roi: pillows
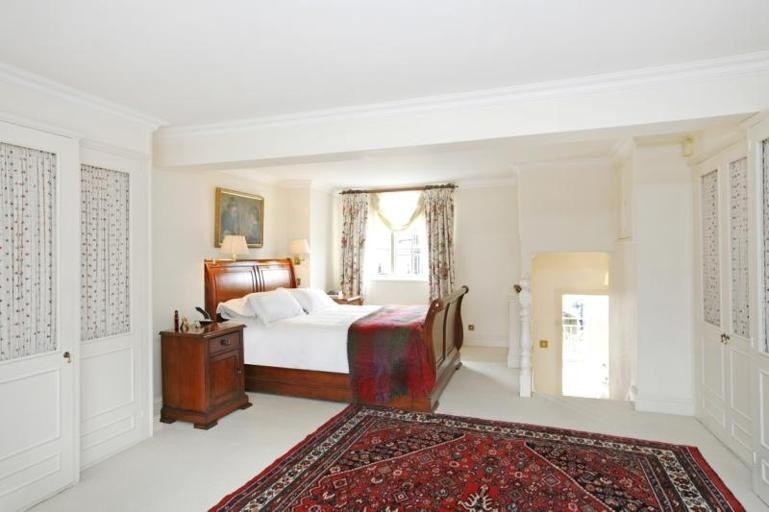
[216,286,338,325]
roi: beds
[204,258,468,412]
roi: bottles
[174,310,189,332]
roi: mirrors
[562,294,610,402]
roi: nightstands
[161,324,249,427]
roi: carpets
[207,401,747,512]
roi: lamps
[288,237,312,265]
[219,234,250,262]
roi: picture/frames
[215,188,264,251]
